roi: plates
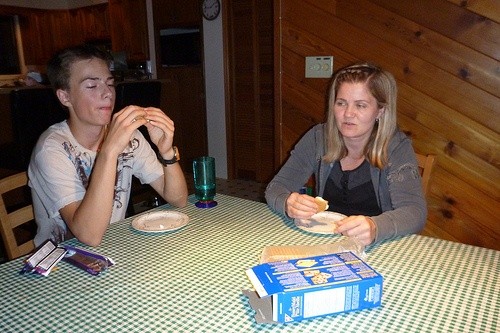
[294,210,349,234]
[131,210,190,233]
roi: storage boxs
[241,250,383,326]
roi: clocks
[200,0,221,21]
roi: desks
[0,79,162,231]
[0,191,500,333]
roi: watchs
[157,146,180,167]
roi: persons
[27,46,188,249]
[265,63,428,247]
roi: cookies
[260,242,359,265]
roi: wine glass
[193,156,218,209]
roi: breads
[313,196,329,213]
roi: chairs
[0,171,39,261]
[412,152,434,197]
